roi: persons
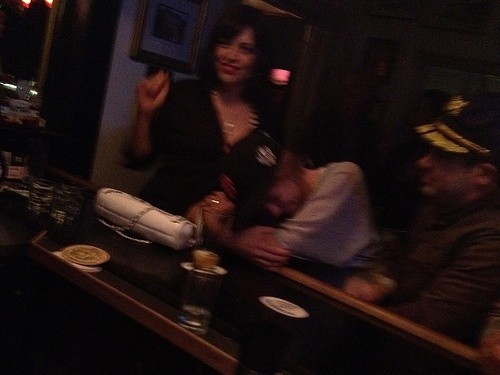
[188,150,396,282]
[120,0,311,245]
[344,96,500,350]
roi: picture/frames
[127,0,210,73]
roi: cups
[194,250,219,270]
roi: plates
[63,243,110,265]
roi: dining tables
[0,171,488,375]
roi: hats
[220,129,281,233]
[410,90,500,156]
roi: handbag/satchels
[92,187,204,250]
